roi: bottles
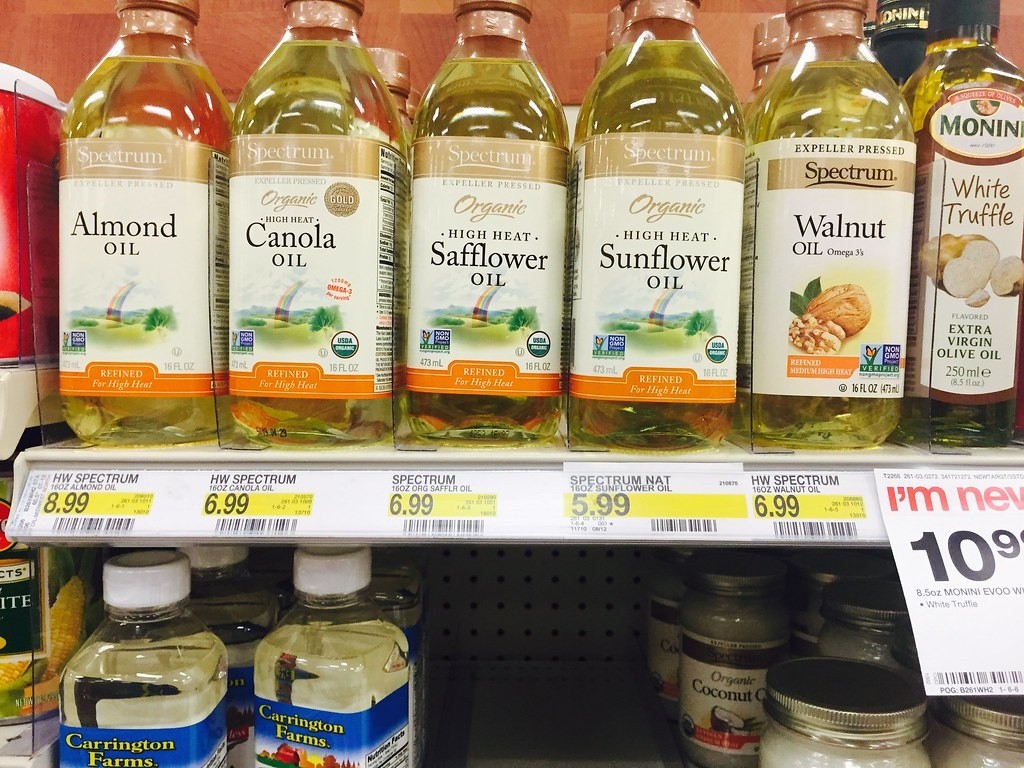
[61,0,1024,768]
